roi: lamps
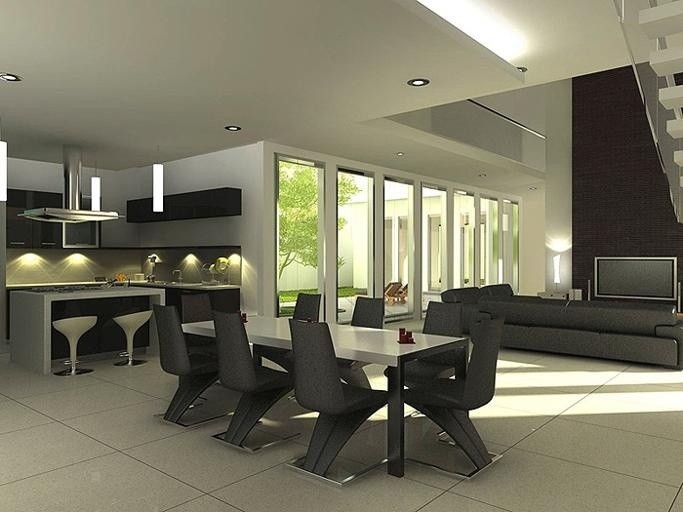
[551,254,562,285]
[152,144,164,212]
[90,159,101,212]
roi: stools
[50,314,99,379]
[110,308,155,368]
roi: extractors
[17,141,125,223]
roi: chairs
[267,293,323,361]
[285,316,388,486]
[383,281,409,305]
[150,304,235,433]
[400,313,506,481]
[338,297,385,389]
[211,311,303,459]
[384,302,464,435]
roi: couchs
[420,284,683,370]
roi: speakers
[574,290,582,300]
[569,289,575,300]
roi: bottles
[237,310,248,324]
[399,327,413,342]
[115,273,123,281]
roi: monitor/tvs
[594,256,678,301]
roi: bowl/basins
[133,274,145,281]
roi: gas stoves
[31,283,105,291]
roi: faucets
[171,269,183,283]
[107,278,118,289]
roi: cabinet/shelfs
[5,188,63,248]
[172,187,241,220]
[126,194,171,223]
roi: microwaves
[61,218,99,249]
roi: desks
[8,288,166,376]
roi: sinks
[169,280,203,287]
